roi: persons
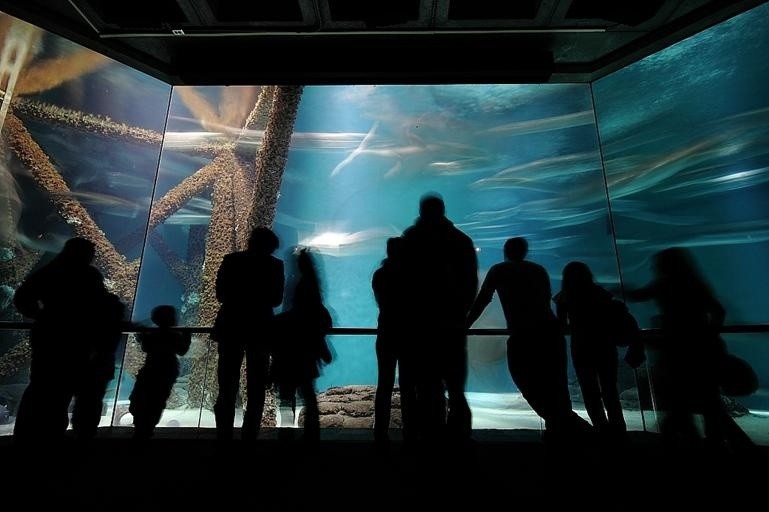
[369,235,411,444]
[70,265,126,435]
[551,262,629,441]
[464,237,597,445]
[398,191,480,454]
[279,248,323,444]
[11,237,99,438]
[609,241,755,446]
[126,304,194,436]
[209,226,288,445]
